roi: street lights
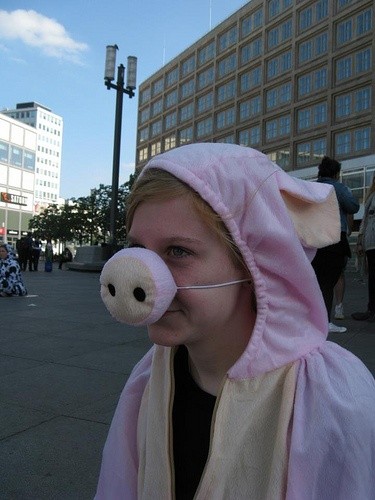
[104,44,138,258]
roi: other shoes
[352,312,373,321]
[335,303,344,319]
[328,322,347,333]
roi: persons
[44,239,55,272]
[353,174,375,321]
[59,247,73,269]
[333,274,345,319]
[0,243,28,296]
[95,140,374,500]
[16,232,42,271]
[311,159,359,333]
